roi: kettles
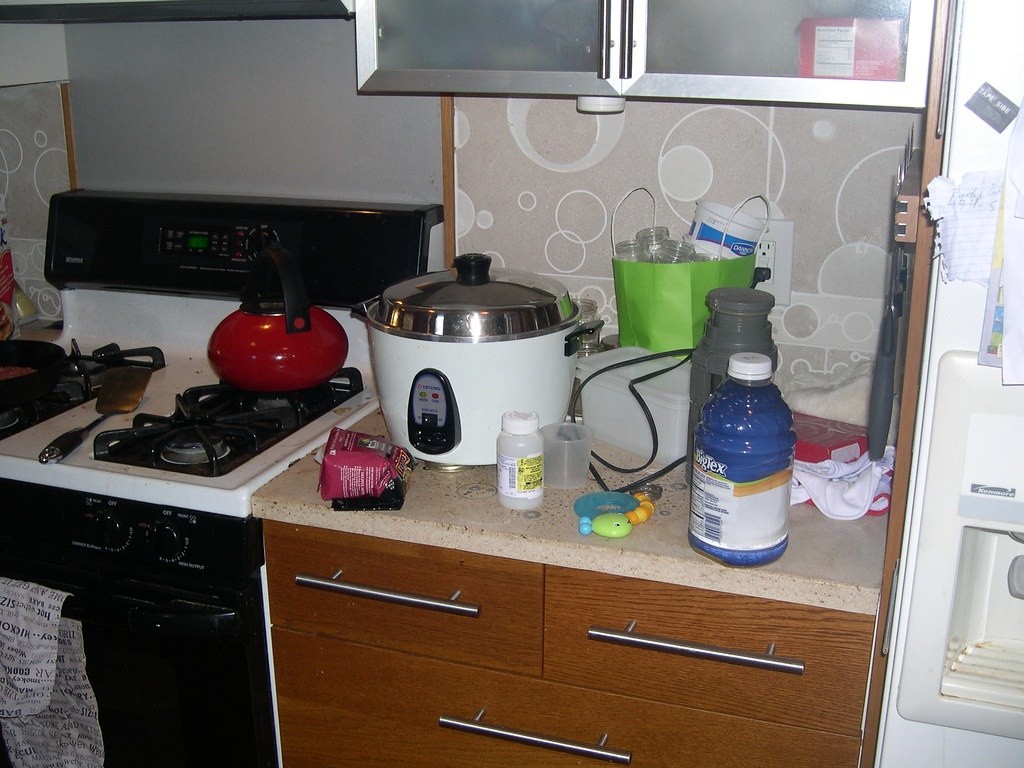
[207,248,349,392]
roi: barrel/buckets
[684,201,768,261]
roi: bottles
[688,352,797,567]
[496,410,544,509]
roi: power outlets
[754,219,796,306]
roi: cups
[615,227,720,265]
[570,298,600,344]
[567,343,604,416]
[540,422,592,489]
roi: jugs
[685,288,777,484]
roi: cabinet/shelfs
[353,0,937,109]
[264,519,876,768]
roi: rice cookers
[350,254,604,473]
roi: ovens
[0,478,278,768]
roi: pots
[0,340,65,403]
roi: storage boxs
[798,18,902,81]
[792,412,869,463]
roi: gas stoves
[0,188,441,518]
